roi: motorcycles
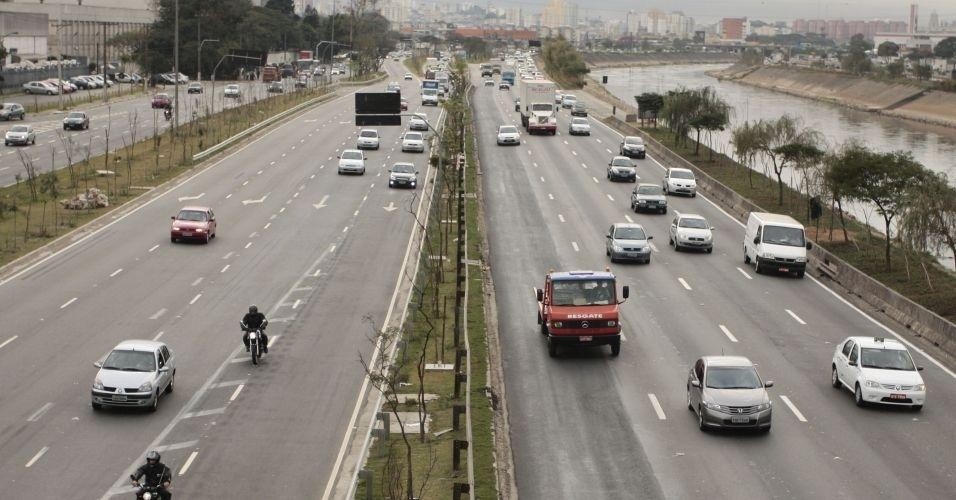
[164,105,171,120]
[238,315,269,365]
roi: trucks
[533,265,632,362]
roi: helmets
[146,449,160,469]
[248,305,257,314]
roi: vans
[743,210,812,278]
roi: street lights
[314,41,339,62]
[95,30,101,73]
[0,31,18,39]
[207,51,263,123]
[55,20,78,112]
[70,31,80,61]
[194,38,222,81]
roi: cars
[5,58,81,73]
[1,101,25,123]
[831,333,929,412]
[150,93,173,109]
[426,52,450,72]
[662,167,699,198]
[670,214,717,254]
[336,71,451,189]
[607,223,654,265]
[629,182,667,217]
[619,133,646,158]
[187,80,203,94]
[90,339,176,410]
[686,354,774,436]
[384,48,411,63]
[63,111,89,132]
[223,83,241,97]
[607,155,636,186]
[170,204,217,246]
[260,54,346,93]
[4,124,37,148]
[477,47,591,144]
[21,70,190,94]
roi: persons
[164,102,172,118]
[256,69,260,79]
[239,304,268,354]
[590,280,610,302]
